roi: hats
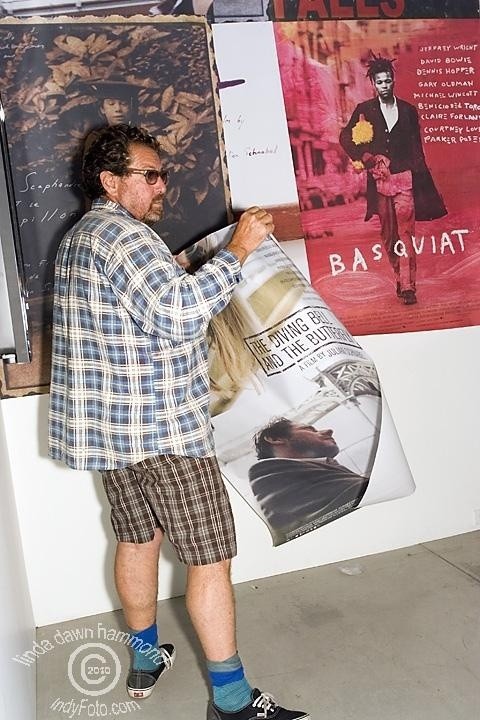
[75,68,148,96]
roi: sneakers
[404,292,417,305]
[208,688,311,719]
[123,642,177,701]
[395,282,402,296]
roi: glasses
[123,164,170,185]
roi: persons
[248,416,369,547]
[338,49,449,304]
[45,122,312,720]
[175,234,257,418]
[76,73,147,125]
